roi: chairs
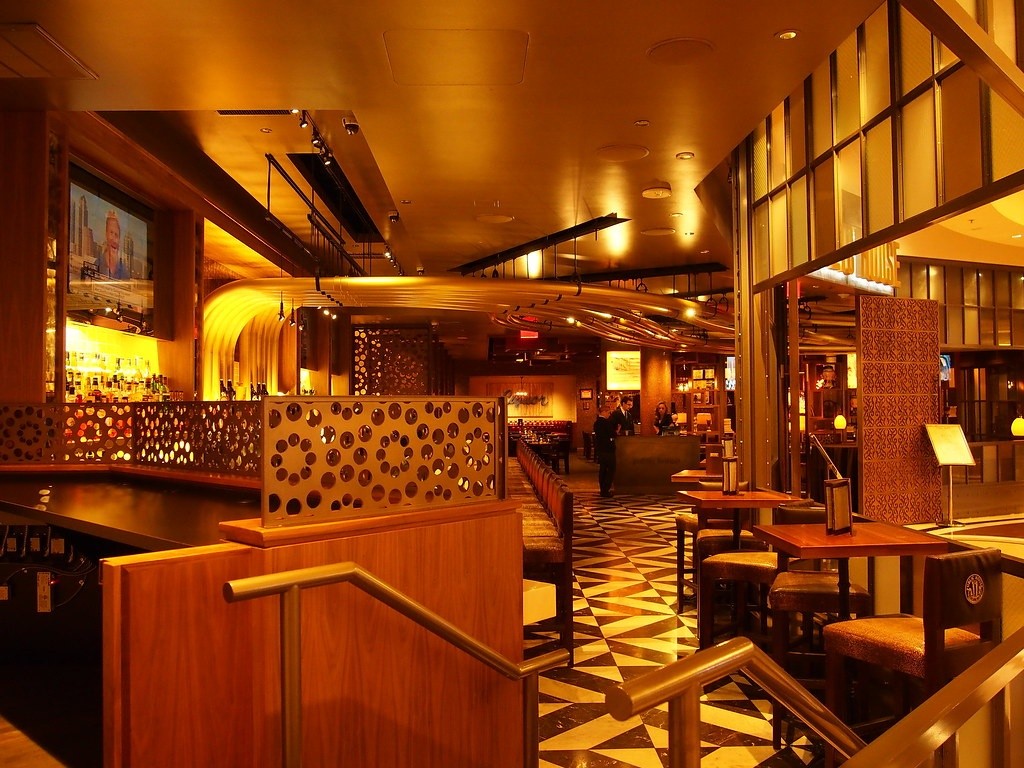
[551,440,570,475]
[582,432,599,463]
[675,481,1004,768]
[507,438,575,667]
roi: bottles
[64,351,184,404]
[233,382,246,401]
[251,382,257,401]
[261,383,268,396]
[220,379,226,400]
[225,380,236,401]
[303,386,316,396]
[256,382,262,401]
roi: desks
[528,441,554,465]
[750,523,949,726]
[677,488,812,643]
[546,434,569,442]
[670,470,723,483]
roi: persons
[517,420,523,430]
[93,217,130,280]
[653,401,674,436]
[610,397,634,430]
[593,404,621,498]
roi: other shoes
[600,490,614,497]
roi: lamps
[676,413,687,426]
[833,415,847,430]
[291,108,332,166]
[278,257,305,331]
[1011,418,1024,436]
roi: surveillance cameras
[341,117,360,134]
[417,266,425,275]
[387,210,398,223]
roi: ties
[625,411,626,421]
